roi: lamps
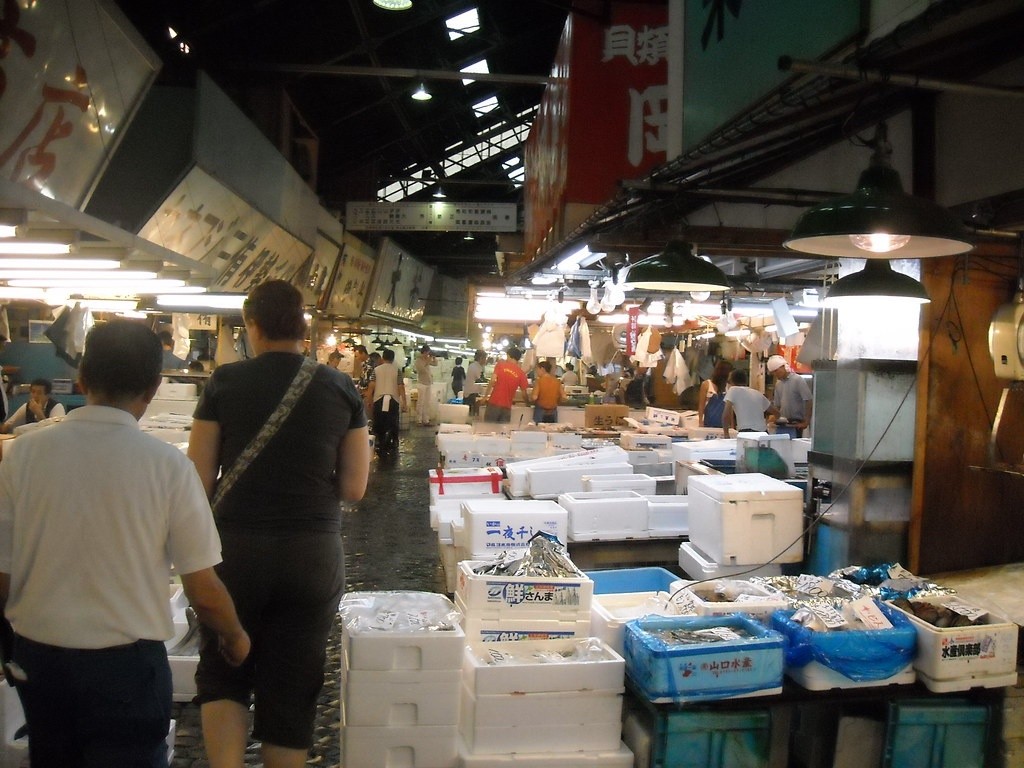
[0,279,212,301]
[369,0,413,13]
[617,210,740,298]
[410,80,433,102]
[0,260,168,280]
[782,130,980,265]
[432,183,447,197]
[826,258,932,304]
[0,227,85,253]
[553,234,608,274]
[0,269,193,289]
[0,246,135,272]
[0,207,33,239]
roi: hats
[767,355,791,372]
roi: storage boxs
[0,382,1024,768]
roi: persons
[451,357,466,398]
[587,366,606,393]
[186,281,370,768]
[529,361,566,425]
[464,350,488,416]
[326,344,383,446]
[0,318,250,768]
[479,348,530,421]
[561,363,579,386]
[366,350,407,448]
[415,345,438,427]
[157,330,188,368]
[171,360,210,396]
[699,355,813,439]
[0,378,65,433]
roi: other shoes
[417,424,422,426]
[424,423,437,426]
